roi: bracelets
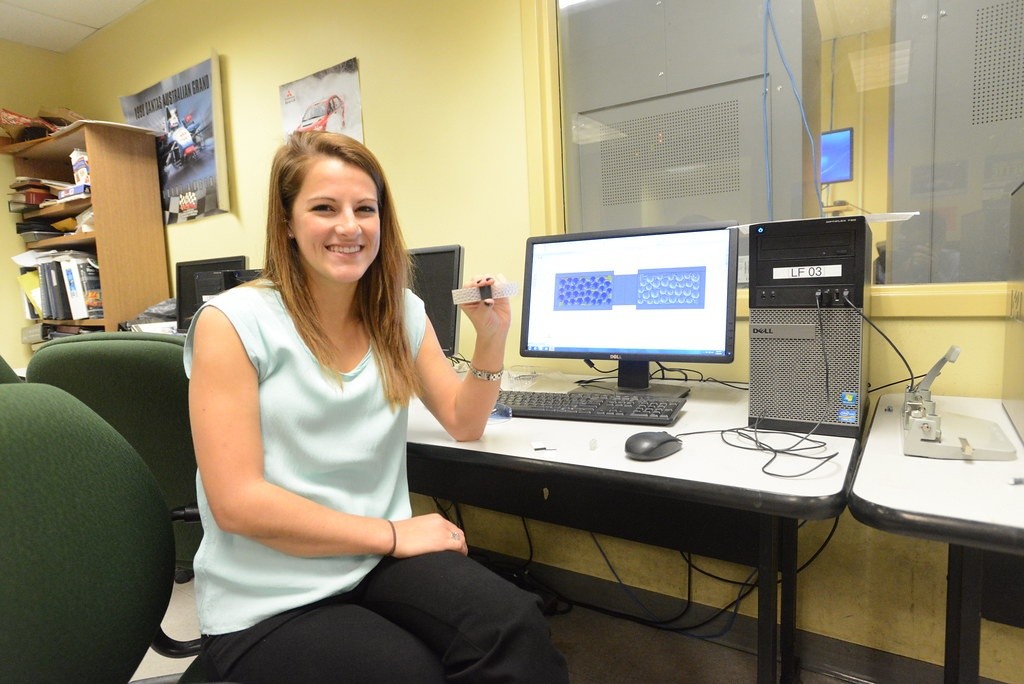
[468,362,508,382]
[383,519,398,556]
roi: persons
[178,126,572,683]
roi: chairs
[0,382,208,684]
[0,331,205,583]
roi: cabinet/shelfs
[11,122,171,352]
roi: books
[77,262,105,320]
[8,178,64,215]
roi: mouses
[625,430,683,461]
[834,201,848,206]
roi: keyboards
[493,390,688,426]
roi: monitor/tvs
[407,244,465,359]
[176,255,246,333]
[820,127,854,184]
[519,220,740,398]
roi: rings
[450,531,459,541]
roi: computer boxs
[196,269,265,309]
[749,216,873,439]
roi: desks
[848,393,1024,684]
[407,368,871,684]
